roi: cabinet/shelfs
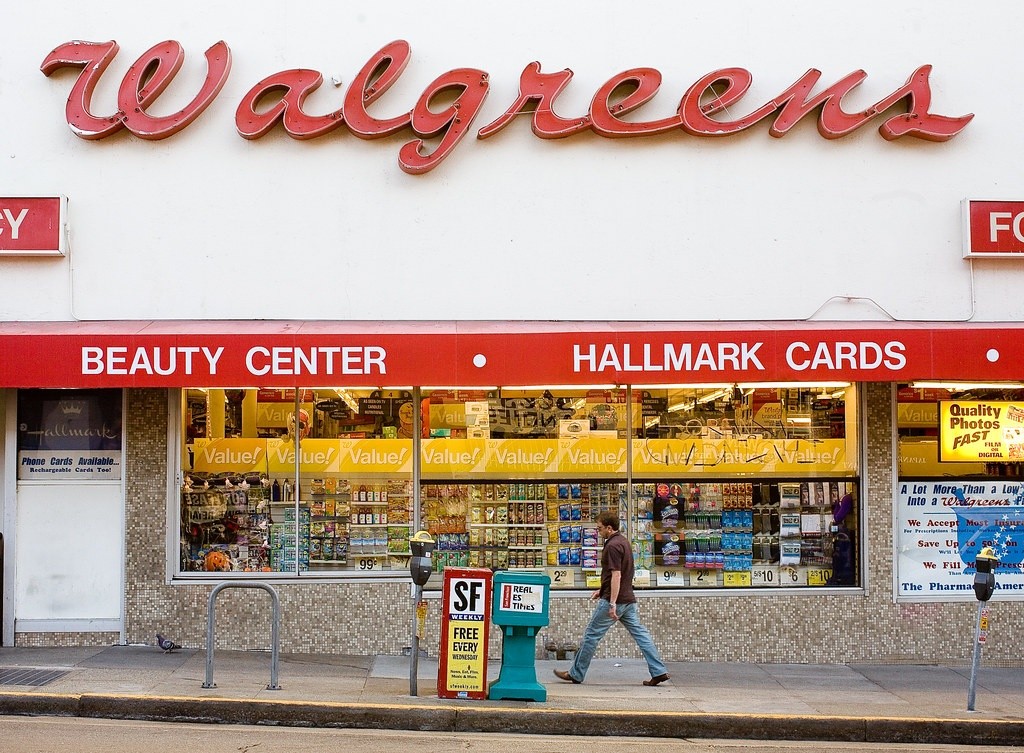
[348,478,654,572]
[309,493,351,563]
[683,482,854,572]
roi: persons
[553,512,671,685]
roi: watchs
[608,603,616,608]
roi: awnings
[0,319,1024,389]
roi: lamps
[668,389,733,413]
[817,387,832,399]
[381,381,852,390]
[910,380,1024,389]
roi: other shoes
[553,669,581,684]
[643,673,670,686]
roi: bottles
[373,482,381,502]
[359,483,367,502]
[352,483,359,502]
[380,483,388,501]
[283,478,290,502]
[273,478,280,501]
[367,483,373,502]
[293,480,300,501]
[351,506,388,525]
[350,528,387,554]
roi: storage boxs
[352,514,387,524]
[326,498,336,516]
[696,553,706,568]
[557,419,617,440]
[465,402,490,439]
[706,553,715,568]
[900,435,985,476]
[430,429,466,439]
[382,426,397,439]
[898,403,939,428]
[429,404,466,428]
[353,491,387,501]
[256,390,316,427]
[337,432,369,438]
[325,477,336,494]
[701,426,763,441]
[270,508,310,572]
[686,552,696,568]
[715,553,724,568]
